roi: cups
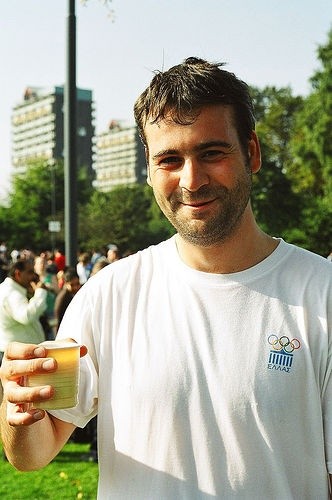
[22,340,80,410]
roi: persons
[0,245,123,462]
[0,57,332,500]
